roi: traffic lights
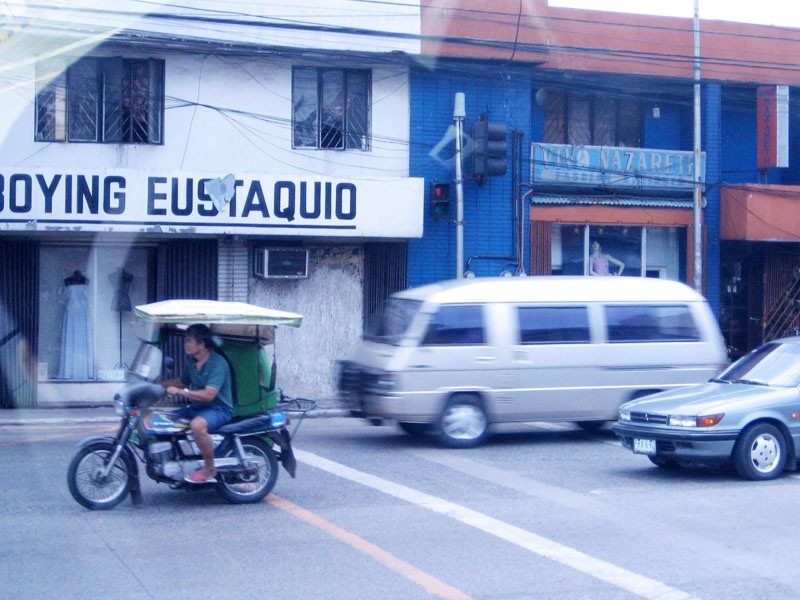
[431,181,451,219]
[473,113,509,177]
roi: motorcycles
[66,298,316,511]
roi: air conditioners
[254,245,309,279]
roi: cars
[612,336,800,480]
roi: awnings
[720,184,800,242]
[529,194,706,226]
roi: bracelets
[184,387,189,396]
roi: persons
[217,338,275,447]
[590,239,625,276]
[301,104,366,148]
[157,325,233,484]
[603,130,626,147]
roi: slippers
[184,471,218,483]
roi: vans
[335,275,727,449]
